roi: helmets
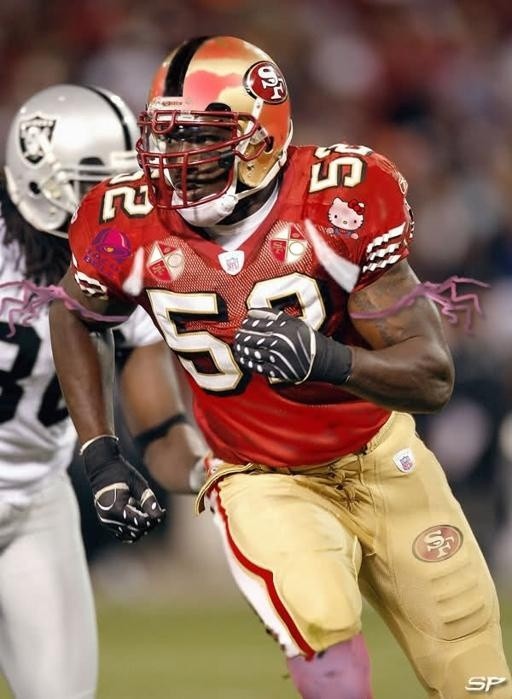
[3,82,140,240]
[134,34,294,228]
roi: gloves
[233,308,350,387]
[80,434,168,545]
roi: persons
[0,80,222,699]
[50,34,512,699]
